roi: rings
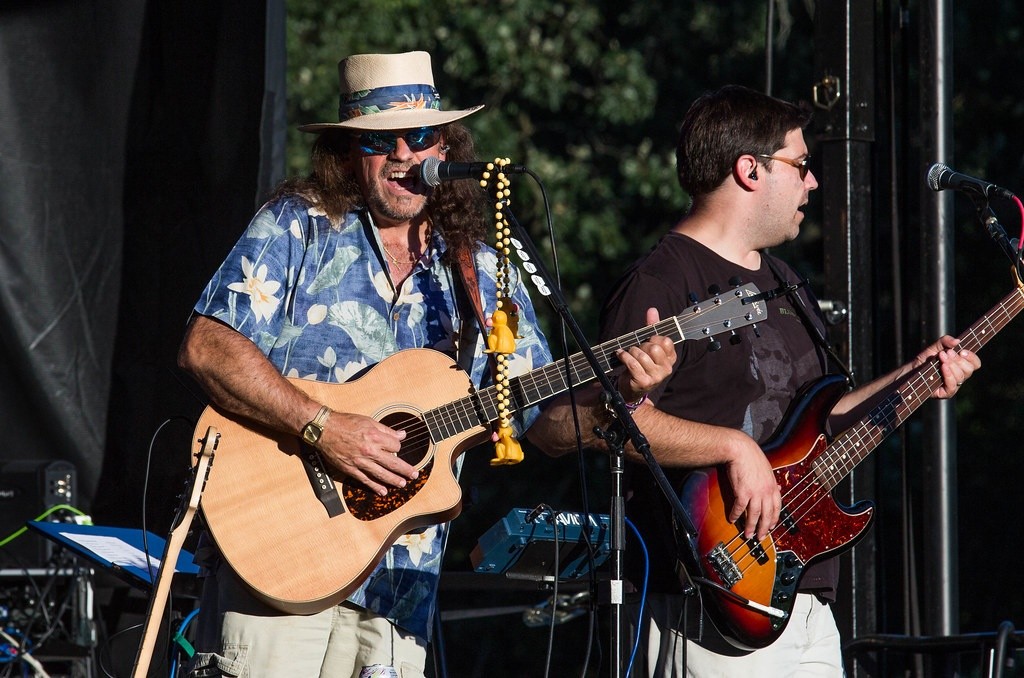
[957,383,964,386]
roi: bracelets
[607,374,648,411]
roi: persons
[594,85,982,678]
[177,77,678,678]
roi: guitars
[672,236,1024,651]
[190,274,810,614]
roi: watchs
[303,406,333,447]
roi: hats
[297,49,487,134]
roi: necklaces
[375,216,434,270]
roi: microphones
[920,162,1016,201]
[419,156,527,189]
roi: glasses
[353,125,443,156]
[744,152,813,182]
